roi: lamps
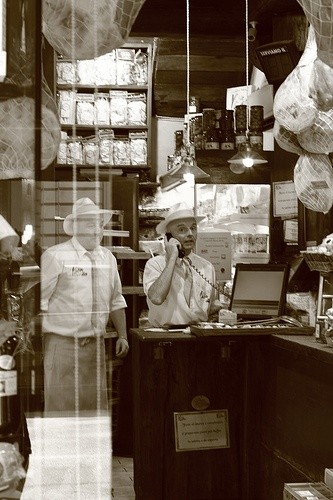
[228,0,268,169]
[170,0,210,180]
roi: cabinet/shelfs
[41,38,159,172]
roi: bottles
[321,294,333,316]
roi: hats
[155,203,206,235]
[63,198,114,239]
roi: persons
[40,197,130,418]
[143,202,217,327]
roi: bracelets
[119,336,127,340]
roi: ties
[86,250,99,327]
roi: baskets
[302,252,333,274]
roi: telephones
[166,232,185,258]
